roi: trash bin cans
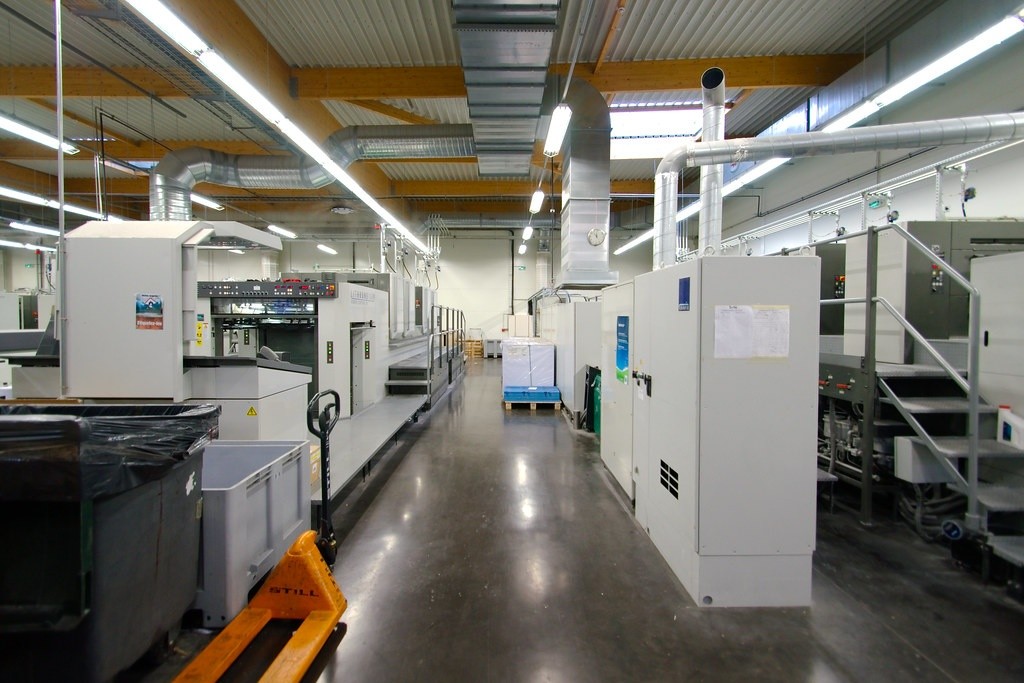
[0,404,223,683]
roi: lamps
[518,99,574,255]
[0,0,429,256]
